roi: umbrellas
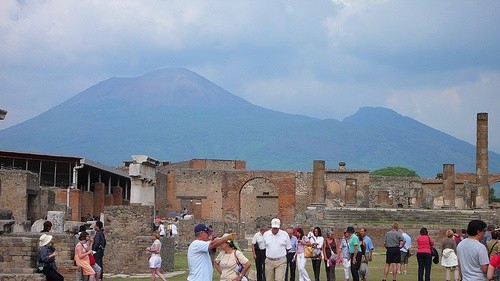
[166,212,178,216]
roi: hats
[194,223,213,233]
[271,218,281,229]
[217,234,232,243]
[39,234,52,246]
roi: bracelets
[238,274,243,279]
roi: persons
[355,228,374,281]
[147,231,167,281]
[440,230,461,281]
[456,220,490,281]
[389,229,412,275]
[452,225,500,281]
[262,218,292,281]
[251,224,269,281]
[285,223,301,281]
[187,223,237,281]
[338,229,353,281]
[347,226,362,281]
[40,221,52,232]
[3,223,11,233]
[308,227,324,281]
[155,207,187,237]
[382,222,406,281]
[323,230,339,281]
[293,228,311,281]
[74,224,102,281]
[416,228,434,281]
[214,233,251,281]
[92,221,107,280]
[37,234,64,281]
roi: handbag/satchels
[234,249,243,272]
[331,254,338,262]
[433,253,439,265]
[89,252,96,266]
[431,247,437,256]
[304,244,315,258]
